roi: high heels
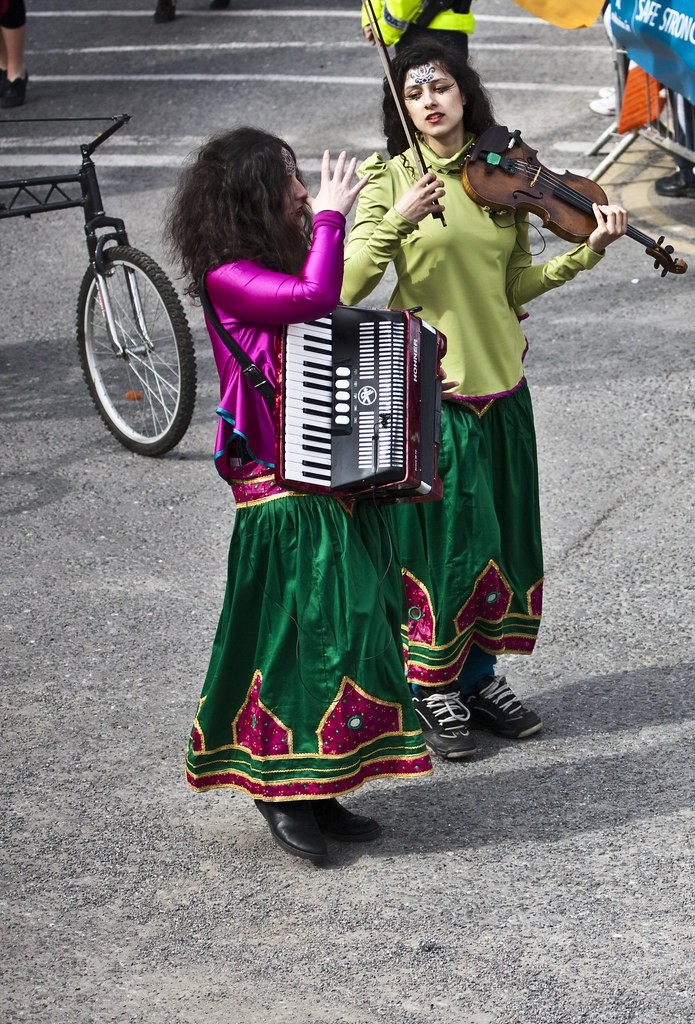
[0,67,28,108]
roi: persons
[163,127,434,862]
[353,0,474,160]
[0,1,29,109]
[344,43,628,760]
[654,88,695,201]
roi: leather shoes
[253,797,327,859]
[313,796,381,841]
[655,172,695,198]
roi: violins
[456,119,693,281]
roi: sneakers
[407,680,477,759]
[465,675,542,737]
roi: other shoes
[153,0,175,24]
[210,0,229,11]
[589,87,616,115]
